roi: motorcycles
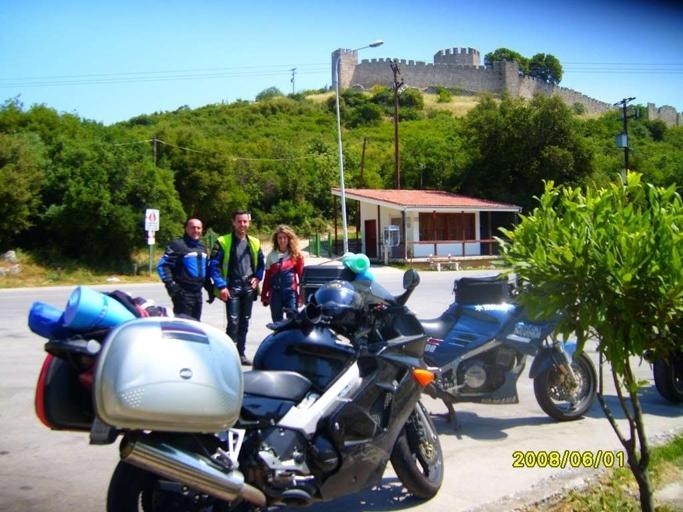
[299,247,598,422]
[26,261,456,512]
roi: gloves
[166,280,184,297]
[204,284,215,304]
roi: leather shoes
[241,356,253,365]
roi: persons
[157,210,303,366]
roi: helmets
[343,256,369,273]
[314,281,364,315]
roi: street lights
[331,40,386,256]
